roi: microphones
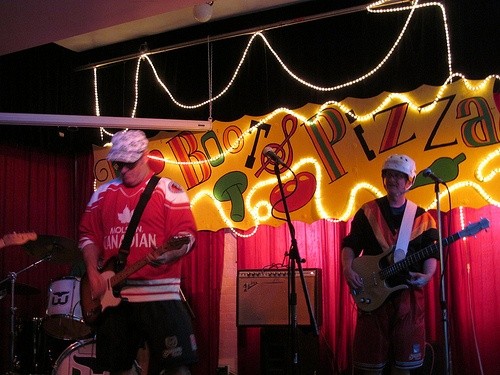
[264,146,286,167]
[423,169,446,186]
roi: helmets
[382,154,416,178]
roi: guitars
[0,231,39,250]
[79,233,192,324]
[350,216,490,311]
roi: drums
[43,273,93,341]
[52,338,141,375]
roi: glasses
[112,159,141,171]
[384,173,407,179]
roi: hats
[107,130,148,163]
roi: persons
[79,128,197,375]
[341,154,439,375]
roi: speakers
[236,267,322,328]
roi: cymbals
[0,276,38,298]
[23,234,80,263]
